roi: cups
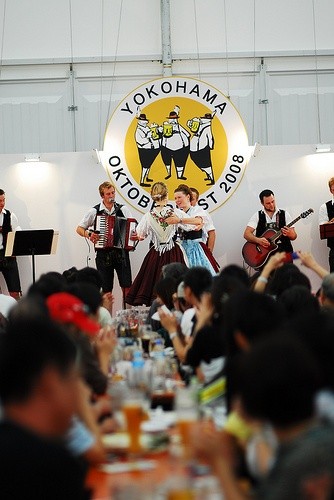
[142,336,150,358]
[151,389,175,412]
[121,400,143,458]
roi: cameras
[292,253,299,259]
[281,253,291,262]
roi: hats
[136,113,149,121]
[167,112,178,119]
[201,114,214,118]
[47,292,102,336]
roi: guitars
[242,208,314,268]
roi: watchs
[170,332,177,341]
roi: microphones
[109,198,116,203]
[89,229,100,234]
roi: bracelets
[258,277,268,284]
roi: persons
[76,181,138,319]
[319,176,334,274]
[125,182,220,308]
[0,250,334,500]
[243,189,297,264]
[0,189,21,302]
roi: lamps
[313,144,330,153]
[24,153,40,162]
